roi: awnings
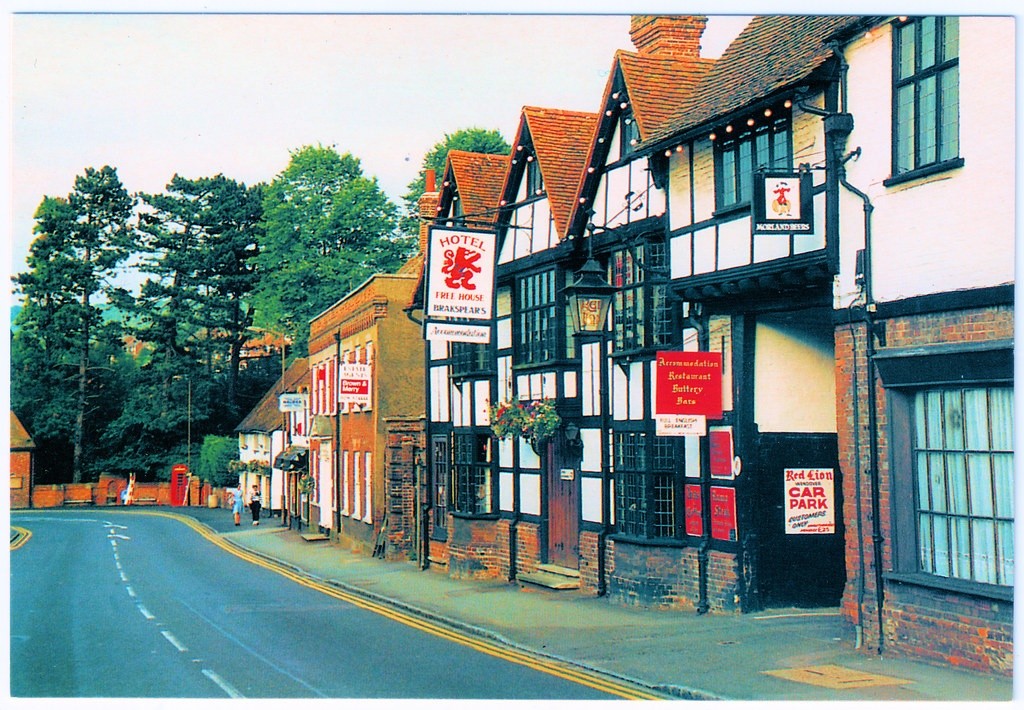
[274,448,308,473]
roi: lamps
[558,208,670,338]
[565,422,582,447]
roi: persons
[231,483,245,526]
[248,485,262,526]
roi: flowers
[227,459,273,473]
[485,393,564,442]
[297,473,315,493]
